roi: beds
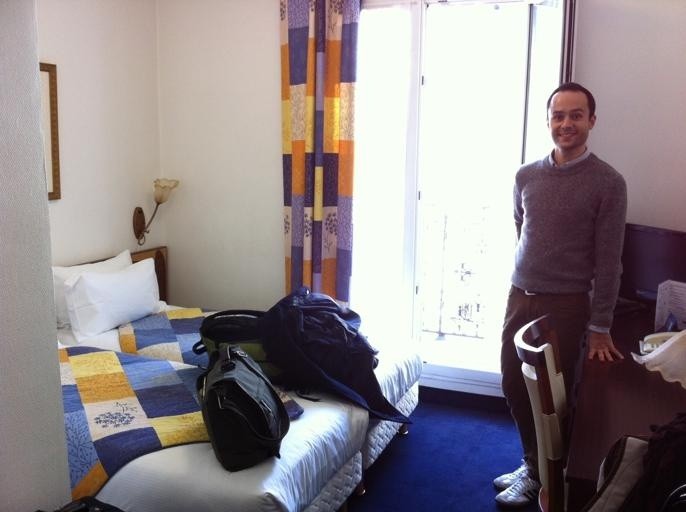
[85,303,424,474]
[59,346,369,512]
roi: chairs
[514,314,572,511]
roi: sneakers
[492,459,540,507]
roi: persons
[492,83,627,506]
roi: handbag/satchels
[579,436,686,511]
[196,345,281,472]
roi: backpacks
[192,309,282,380]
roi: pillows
[52,247,160,343]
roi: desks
[573,299,686,511]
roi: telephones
[643,332,686,352]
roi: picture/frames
[39,62,60,201]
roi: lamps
[134,179,179,246]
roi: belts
[524,289,538,296]
